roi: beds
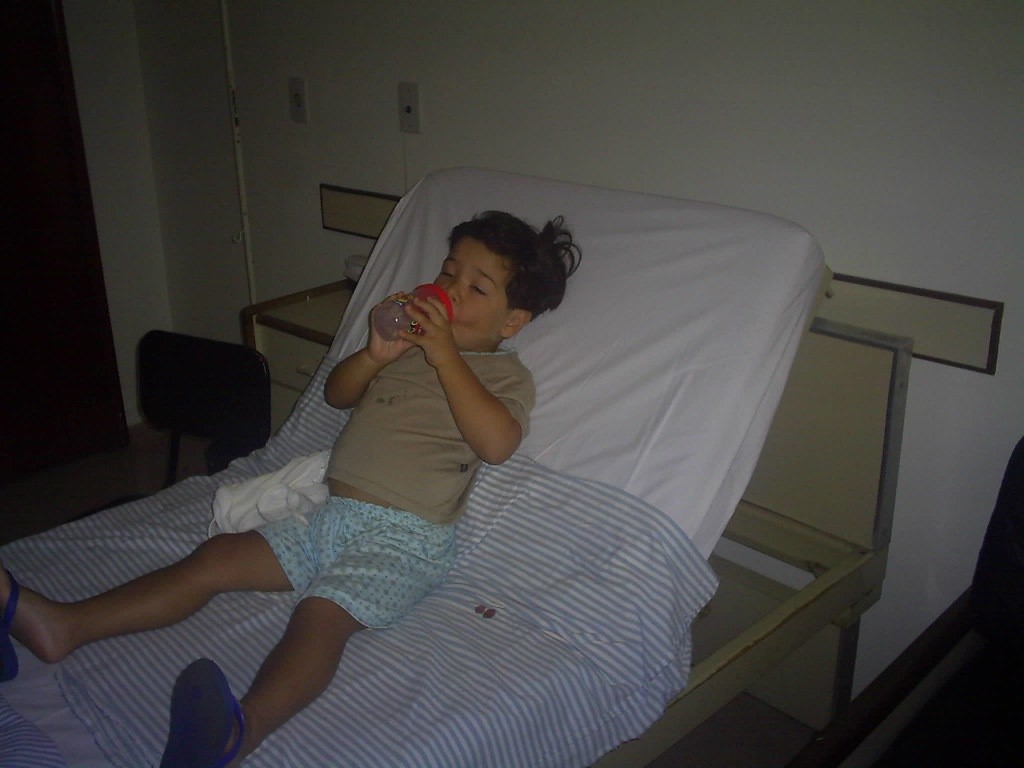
[0,165,915,768]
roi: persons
[0,208,583,767]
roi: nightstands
[240,278,356,439]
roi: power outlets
[398,82,422,135]
[288,78,310,124]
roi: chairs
[69,330,272,528]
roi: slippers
[0,568,19,681]
[160,655,244,768]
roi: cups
[373,285,453,342]
